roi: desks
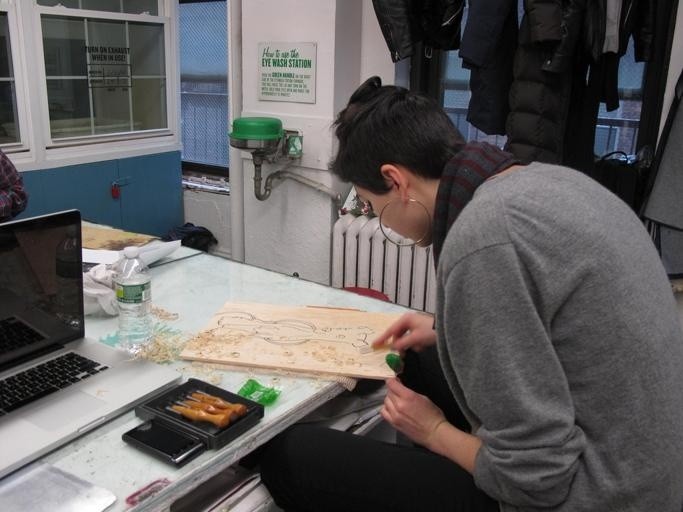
[1,227,431,511]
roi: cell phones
[121,420,202,465]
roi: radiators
[330,214,441,315]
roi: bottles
[114,246,152,343]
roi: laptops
[0,207,184,479]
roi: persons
[258,74,683,512]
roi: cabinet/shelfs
[46,136,185,236]
